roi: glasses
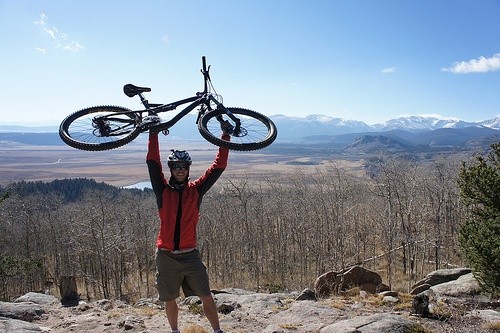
[169,164,189,171]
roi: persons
[146,119,235,333]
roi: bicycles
[58,56,278,152]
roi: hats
[168,150,192,162]
